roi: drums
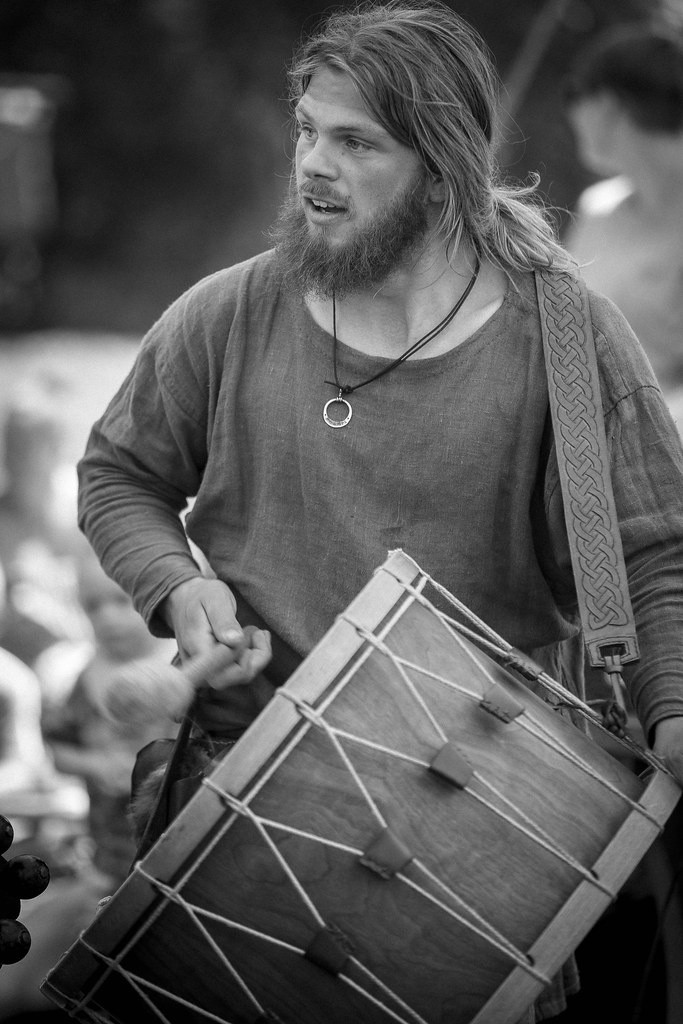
[34,545,683,1024]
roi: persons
[565,36,683,432]
[76,8,682,1024]
[1,408,195,888]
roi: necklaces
[323,238,481,429]
